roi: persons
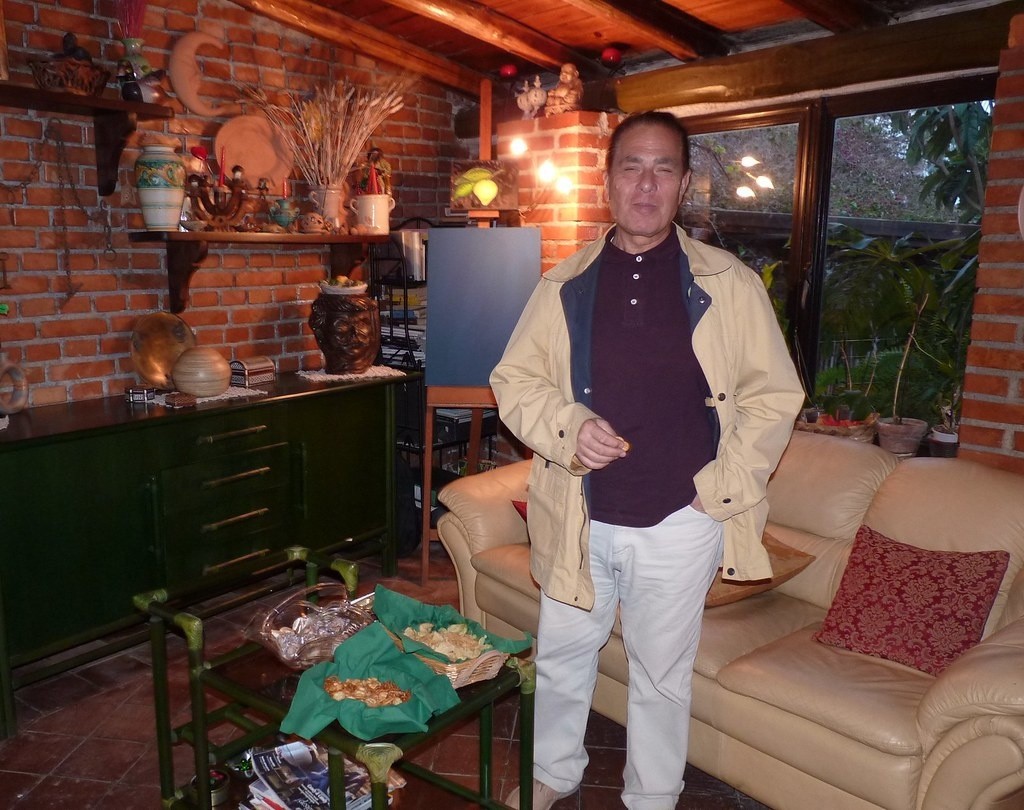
[487,111,806,810]
[547,64,583,104]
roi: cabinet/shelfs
[371,219,494,556]
[0,366,423,739]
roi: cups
[349,194,396,235]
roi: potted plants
[790,286,961,460]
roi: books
[435,409,496,422]
[379,229,428,282]
[381,286,426,367]
[438,207,499,228]
[237,741,407,810]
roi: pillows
[707,531,817,608]
[511,499,527,520]
[813,524,1010,677]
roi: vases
[311,186,344,229]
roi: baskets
[381,623,510,689]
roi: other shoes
[505,779,580,810]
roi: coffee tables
[129,547,540,810]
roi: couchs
[437,428,1024,810]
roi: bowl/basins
[128,312,197,391]
